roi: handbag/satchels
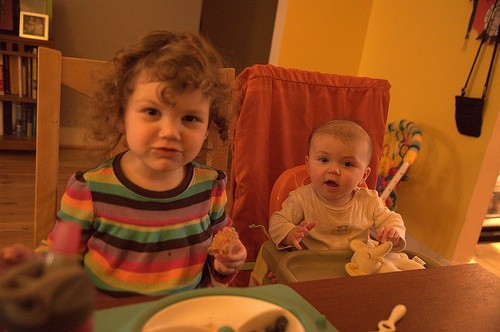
[454,94,485,138]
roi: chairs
[33,46,235,251]
[224,63,444,287]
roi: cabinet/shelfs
[0,34,54,152]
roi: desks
[76,262,500,332]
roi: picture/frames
[18,10,50,42]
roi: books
[0,101,36,138]
[0,54,10,95]
[23,48,37,101]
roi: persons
[27,17,42,34]
[268,120,406,251]
[0,30,248,295]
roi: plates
[141,296,305,332]
